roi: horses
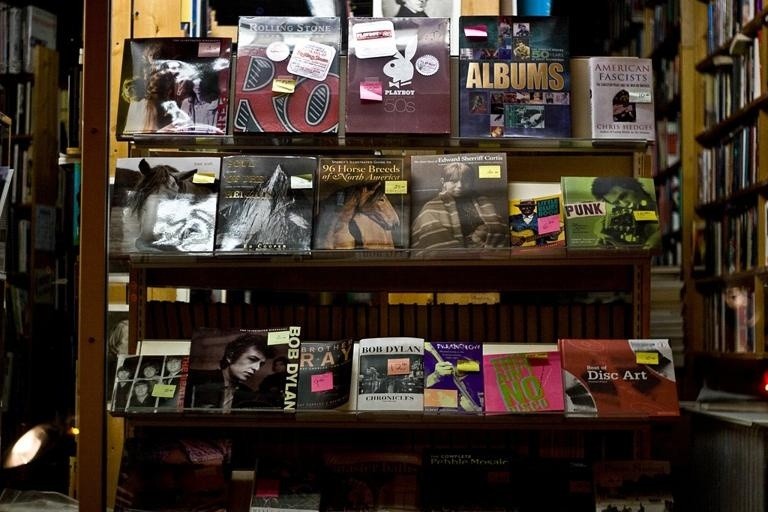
[126,158,219,254]
[315,180,401,251]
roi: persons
[113,332,288,408]
[410,161,508,255]
[128,165,170,253]
[120,42,231,137]
[509,195,562,247]
[591,176,661,250]
[470,23,570,137]
[394,0,429,17]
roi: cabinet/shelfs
[0,1,767,510]
